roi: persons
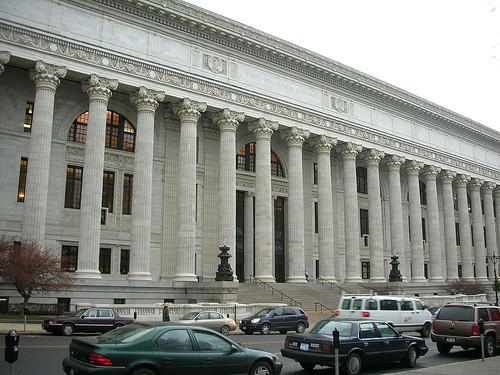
[305,270,309,282]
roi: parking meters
[478,320,485,361]
[134,312,137,320]
[332,327,341,375]
[24,306,28,331]
[5,329,20,375]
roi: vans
[335,294,434,338]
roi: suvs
[431,301,500,357]
[238,306,310,335]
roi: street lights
[485,251,500,307]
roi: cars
[41,306,135,337]
[280,317,430,375]
[62,320,284,375]
[175,311,237,336]
